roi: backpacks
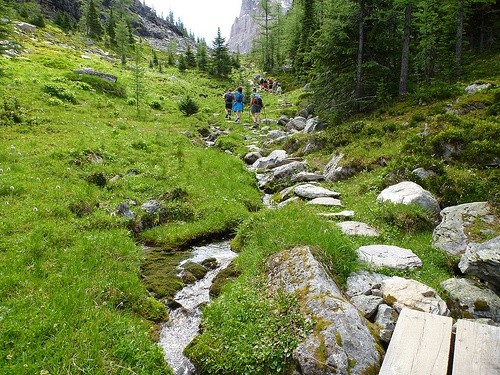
[236,92,244,102]
[253,94,262,106]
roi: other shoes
[252,123,256,128]
[235,116,239,122]
[229,116,231,120]
[237,120,240,123]
[256,125,259,129]
[225,113,229,118]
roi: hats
[252,87,256,91]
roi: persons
[232,86,245,123]
[222,88,235,120]
[303,83,310,93]
[247,87,262,129]
[252,73,282,96]
